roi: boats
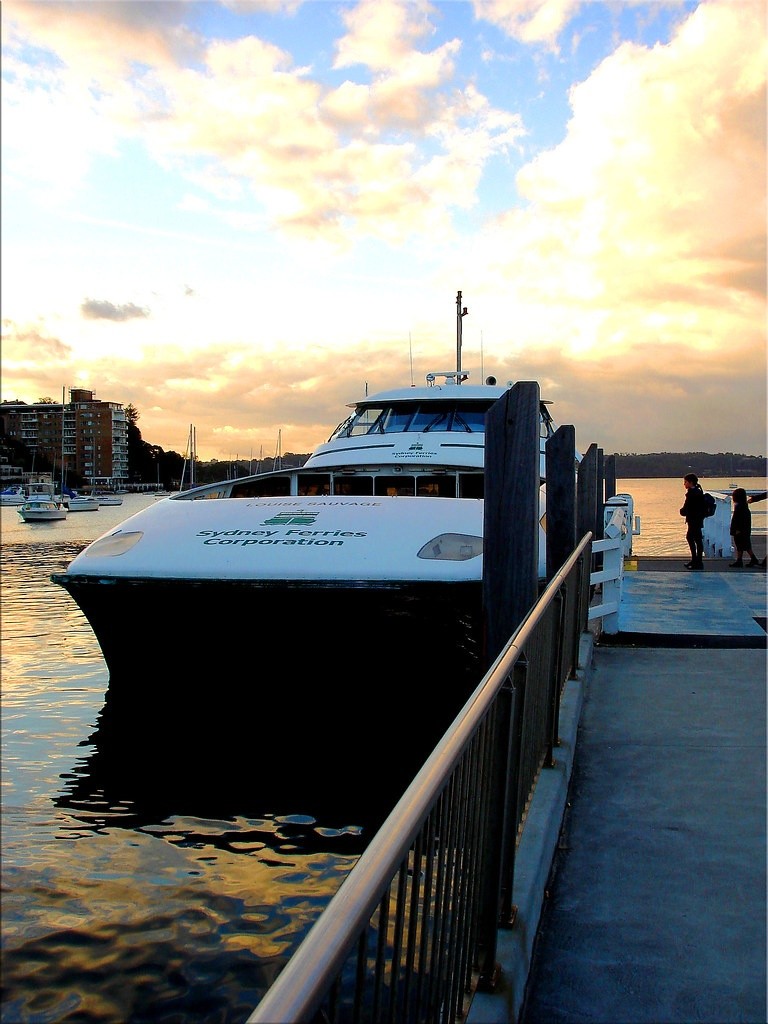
[17,492,68,523]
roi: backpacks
[698,489,717,517]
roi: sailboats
[728,457,738,488]
[0,384,124,511]
[153,463,171,497]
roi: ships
[45,287,583,711]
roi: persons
[680,474,705,569]
[748,493,768,567]
[729,489,758,567]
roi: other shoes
[687,564,704,570]
[728,562,743,567]
[684,563,689,568]
[745,559,759,567]
